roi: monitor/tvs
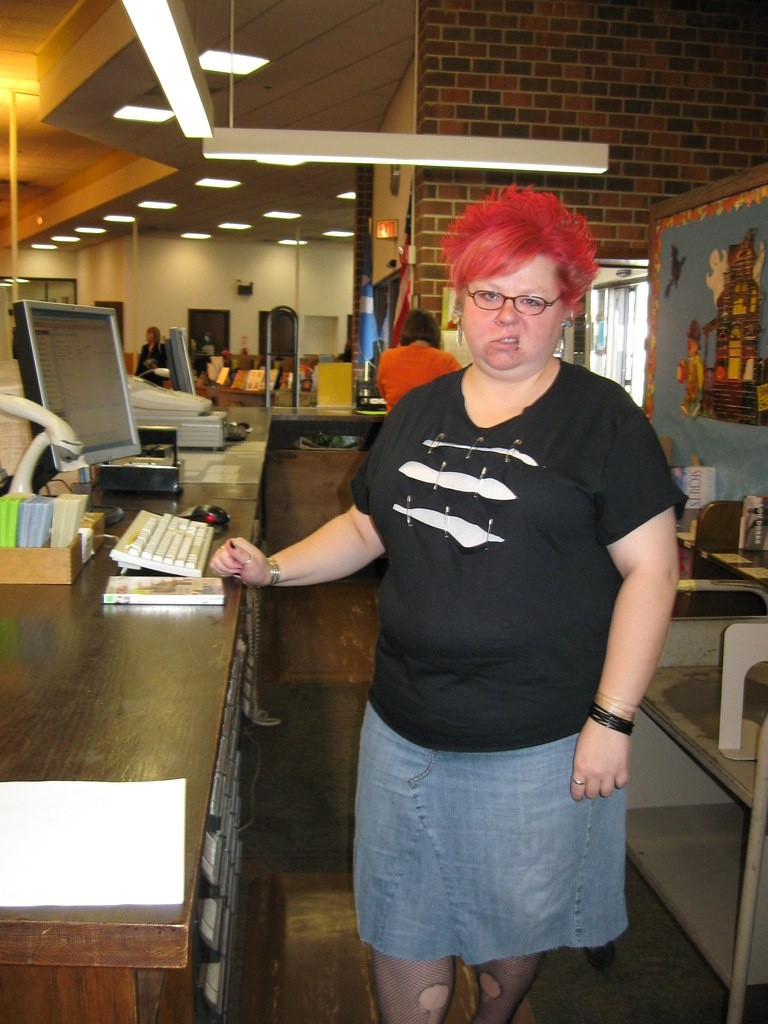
[7,299,142,499]
[165,327,196,395]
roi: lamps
[123,0,215,139]
[203,127,608,174]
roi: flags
[392,193,414,349]
[358,231,378,366]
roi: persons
[208,185,694,1024]
[378,308,463,412]
[134,327,170,389]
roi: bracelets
[593,690,637,722]
[589,701,634,737]
[266,557,281,586]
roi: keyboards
[228,425,248,442]
[110,510,215,578]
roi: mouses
[231,421,250,429]
[177,505,230,525]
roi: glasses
[465,282,563,315]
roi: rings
[572,778,585,785]
[221,546,226,550]
[246,554,252,564]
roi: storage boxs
[1,512,106,584]
